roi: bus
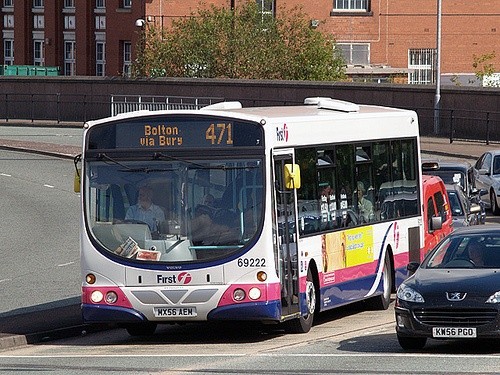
[73,97,425,340]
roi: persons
[357,181,373,221]
[124,186,165,240]
[468,241,490,266]
[203,193,215,207]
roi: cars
[394,222,500,354]
[421,159,488,229]
[473,150,500,217]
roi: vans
[420,172,454,268]
